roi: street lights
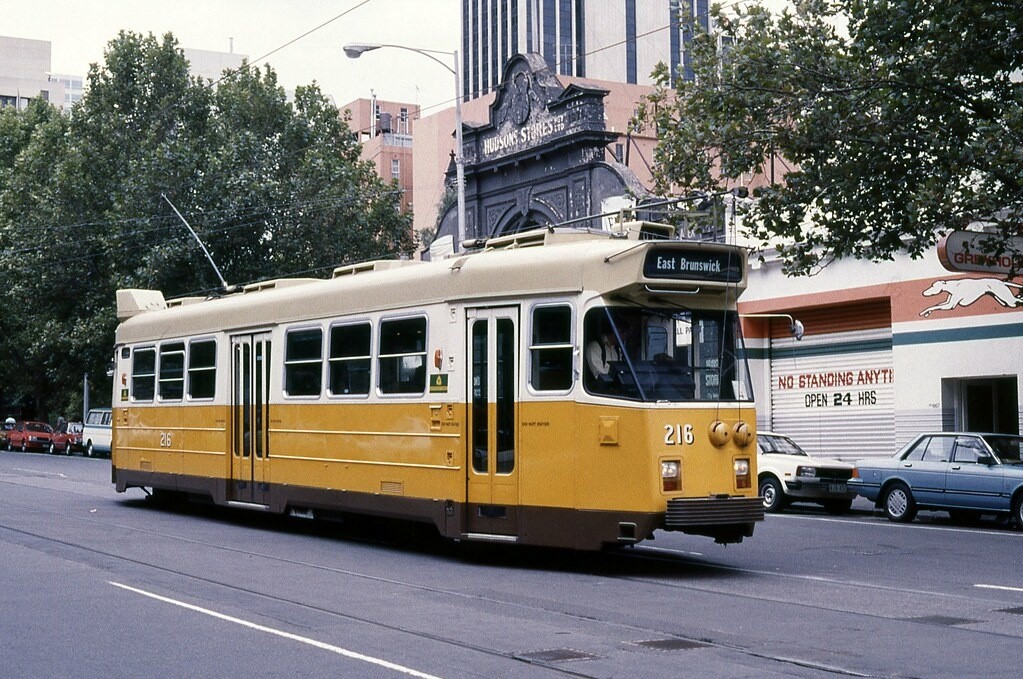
[343,41,466,253]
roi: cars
[753,431,858,516]
[847,431,1023,532]
[5,421,55,452]
[48,421,83,455]
[0,417,18,450]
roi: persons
[57,412,66,427]
[5,414,16,429]
[585,320,634,393]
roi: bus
[109,186,804,557]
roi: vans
[82,408,113,457]
